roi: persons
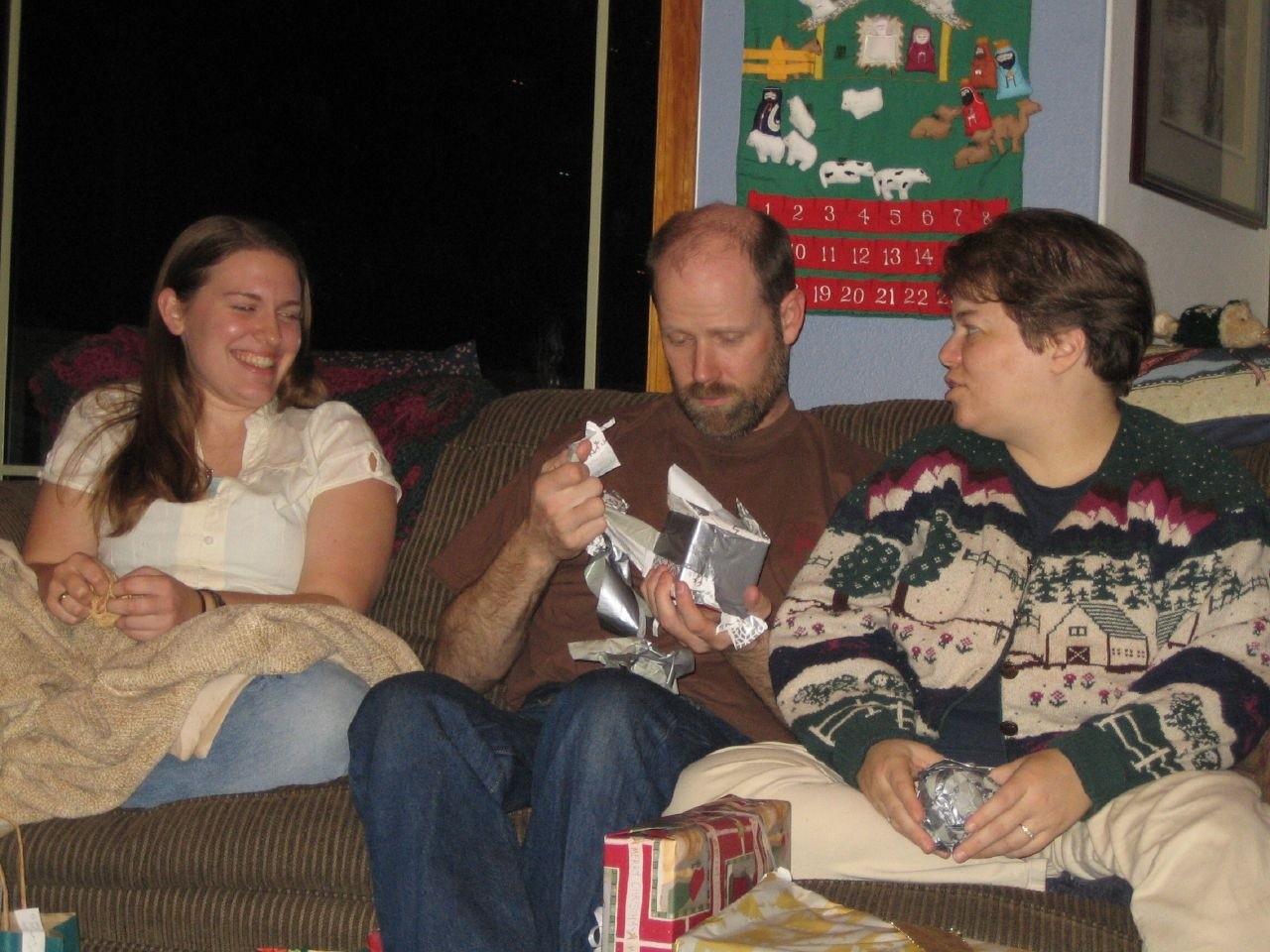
[349,199,886,952]
[663,208,1270,952]
[20,217,404,809]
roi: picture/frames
[1129,0,1270,227]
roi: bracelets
[198,590,206,613]
[201,588,224,608]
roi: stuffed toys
[1154,298,1270,348]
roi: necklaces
[206,469,213,478]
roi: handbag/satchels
[0,814,80,952]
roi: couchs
[0,390,1270,952]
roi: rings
[58,592,69,604]
[1020,823,1035,840]
[887,816,892,823]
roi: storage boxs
[650,505,772,616]
[599,791,796,952]
